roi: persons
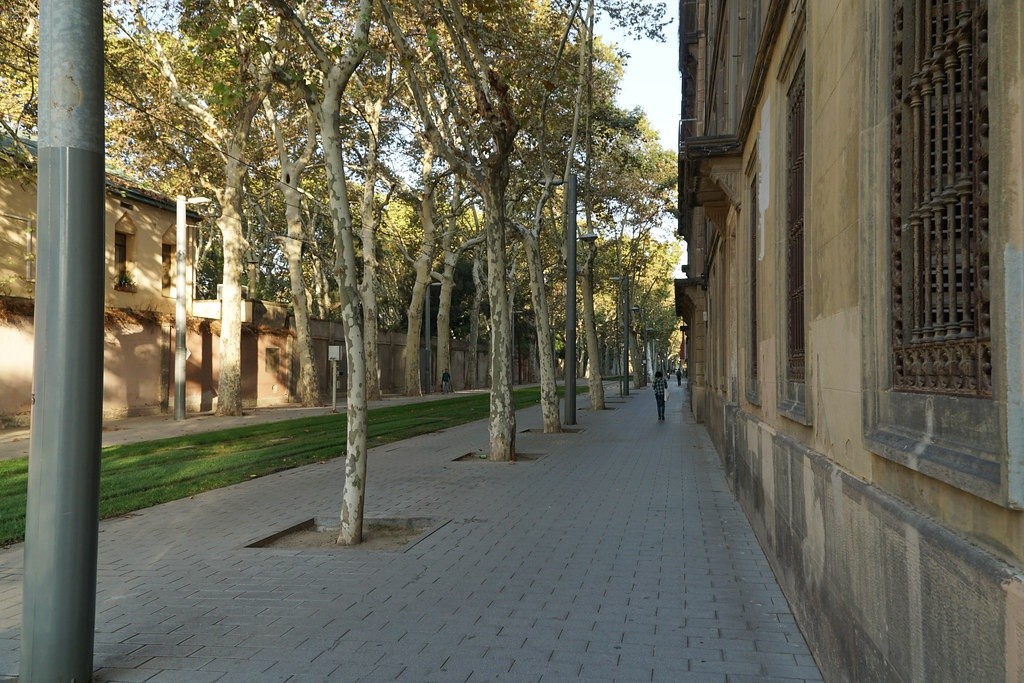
[676,369,682,386]
[442,369,450,394]
[652,371,670,421]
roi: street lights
[173,194,211,418]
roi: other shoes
[658,418,661,420]
[662,418,664,420]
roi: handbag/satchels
[664,389,669,401]
